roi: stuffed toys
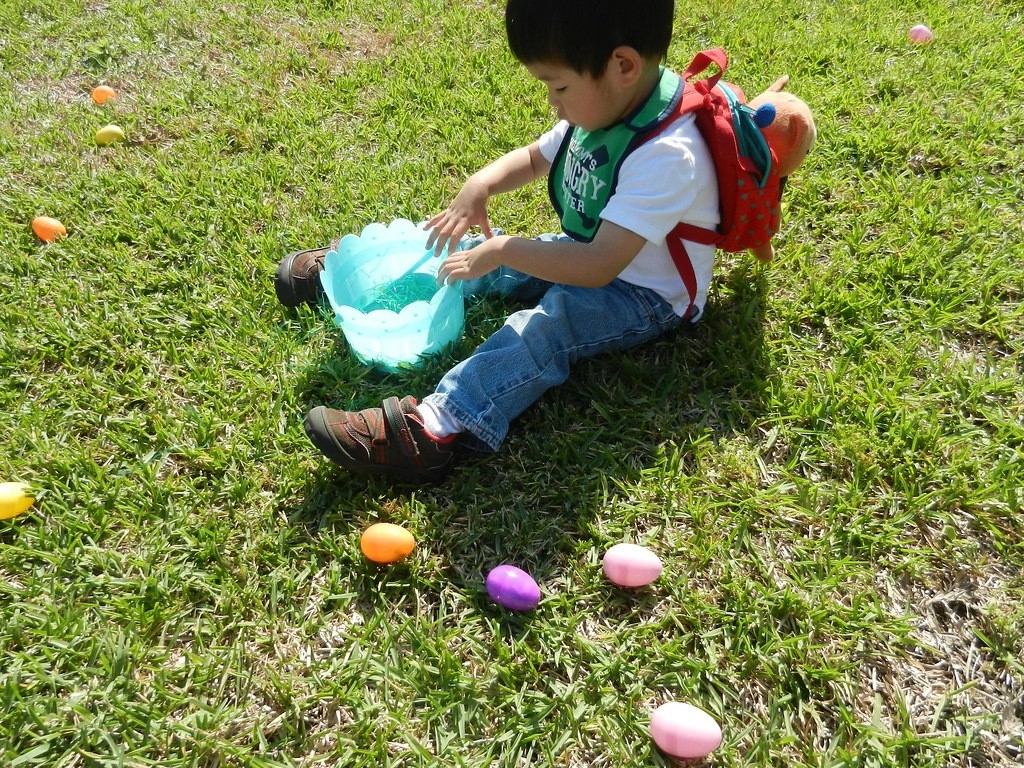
[747,72,818,289]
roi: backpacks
[621,48,817,262]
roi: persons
[274,0,819,486]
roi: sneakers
[276,246,341,309]
[303,395,458,484]
